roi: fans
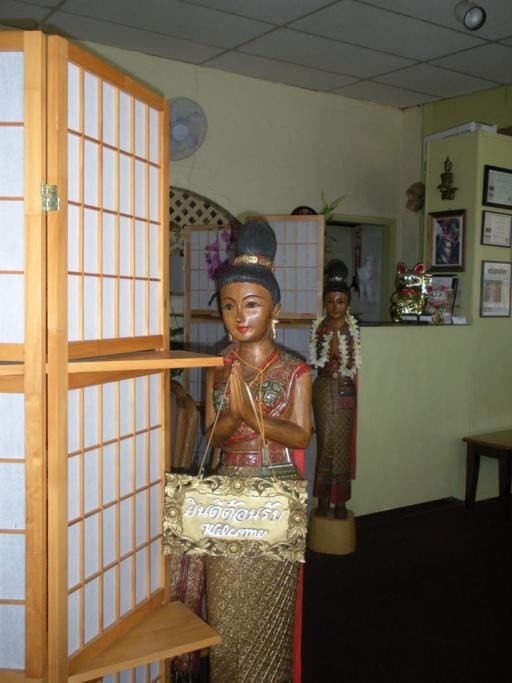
[168,96,209,161]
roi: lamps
[455,1,486,31]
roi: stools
[463,428,511,522]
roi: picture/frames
[478,163,511,318]
[425,208,467,272]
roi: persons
[312,257,360,519]
[436,219,459,265]
[203,219,313,683]
